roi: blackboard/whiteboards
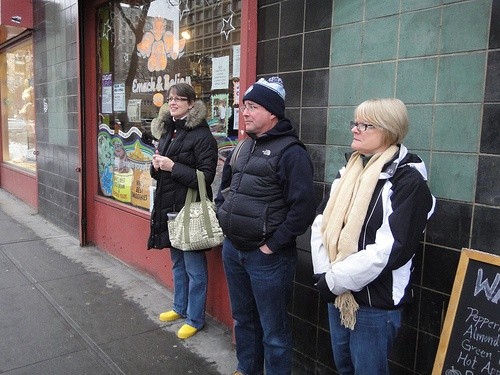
[431,246,500,375]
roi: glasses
[350,120,375,132]
[167,96,188,102]
[240,105,255,114]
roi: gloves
[312,272,336,297]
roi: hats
[242,76,286,119]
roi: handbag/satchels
[167,169,225,252]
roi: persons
[18,91,35,151]
[214,76,315,375]
[147,83,218,338]
[311,98,436,375]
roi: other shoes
[160,310,181,321]
[177,323,198,339]
